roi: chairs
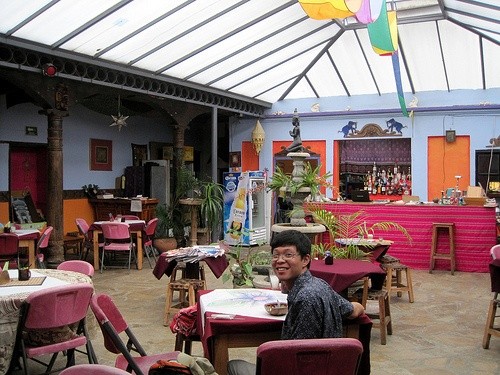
[483,244,500,348]
[0,215,191,375]
[255,337,365,375]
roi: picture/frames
[90,138,112,171]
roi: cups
[19,268,31,281]
[325,256,333,264]
[367,234,383,243]
[4,226,11,233]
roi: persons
[286,117,303,152]
[230,231,368,375]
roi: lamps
[42,62,57,77]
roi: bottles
[225,172,249,245]
[438,181,464,206]
[325,251,331,258]
[363,162,411,196]
[117,215,122,223]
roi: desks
[196,290,371,375]
[0,269,94,375]
[152,244,228,308]
[0,232,39,269]
[179,199,205,248]
[93,195,158,270]
[309,238,393,293]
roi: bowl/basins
[462,196,488,206]
[265,302,288,316]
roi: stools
[429,222,456,276]
[162,263,206,327]
[346,254,414,345]
[176,332,200,354]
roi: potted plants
[217,224,270,288]
[148,147,223,254]
[269,161,333,201]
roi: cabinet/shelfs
[475,148,500,198]
[341,171,364,196]
[272,156,321,223]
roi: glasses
[271,253,301,260]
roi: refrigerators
[224,171,267,247]
